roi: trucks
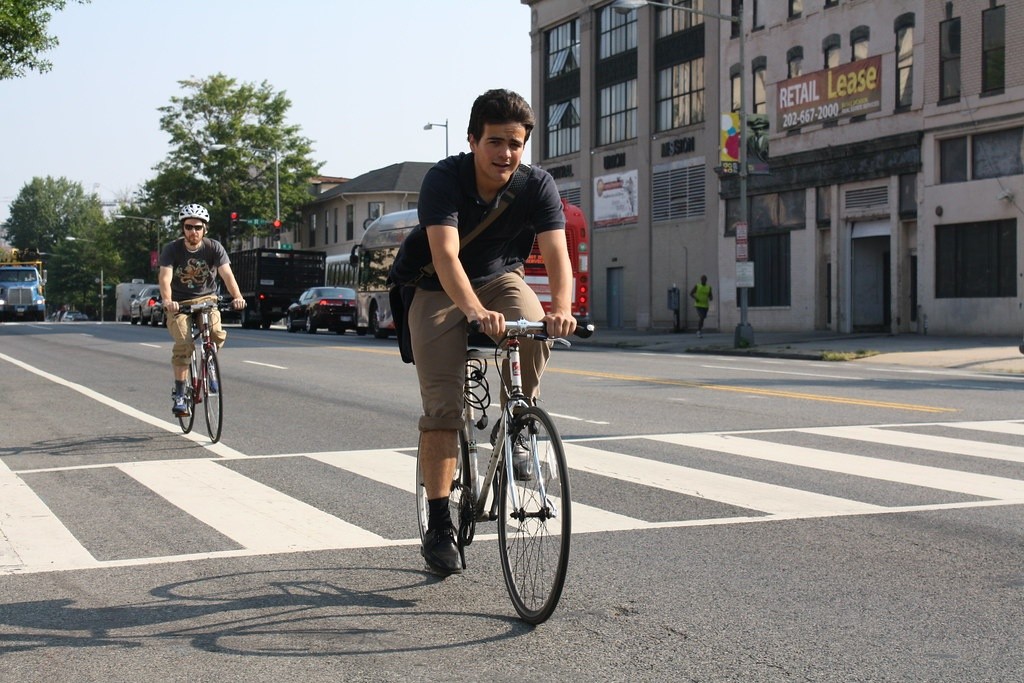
[217,246,327,330]
[0,248,48,322]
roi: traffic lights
[273,219,282,241]
[230,211,240,235]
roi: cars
[48,308,89,322]
[130,284,161,325]
[286,286,357,335]
[149,292,167,328]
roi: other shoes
[696,329,702,338]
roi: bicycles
[164,295,248,444]
[415,314,595,625]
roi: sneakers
[172,395,188,415]
[205,365,220,393]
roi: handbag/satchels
[387,277,418,365]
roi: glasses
[181,224,205,231]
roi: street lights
[424,118,449,159]
[209,143,282,249]
[65,236,104,322]
[610,0,755,350]
[114,214,161,271]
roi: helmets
[178,204,210,223]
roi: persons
[156,204,246,414]
[747,118,769,161]
[407,89,578,575]
[24,273,35,282]
[690,274,713,338]
[47,304,52,319]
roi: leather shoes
[421,526,463,575]
[490,417,536,481]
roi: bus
[349,196,597,340]
[323,253,360,288]
